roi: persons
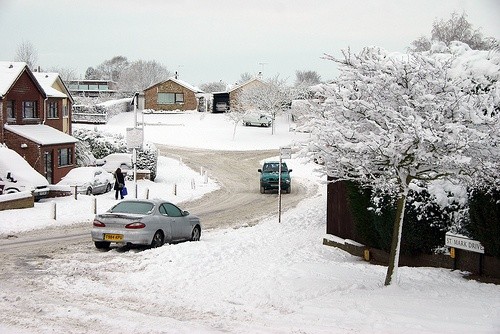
[266,164,272,170]
[114,168,125,200]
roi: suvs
[216,102,229,112]
[258,160,292,194]
[243,114,271,127]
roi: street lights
[126,92,144,182]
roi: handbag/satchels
[119,183,124,188]
[121,187,127,195]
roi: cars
[56,166,115,196]
[90,198,202,249]
